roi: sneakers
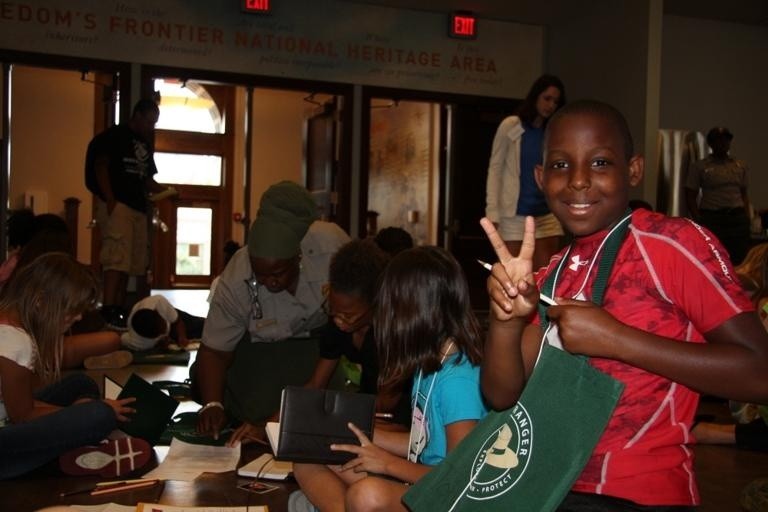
[83,350,132,369]
[101,304,127,331]
[59,439,153,480]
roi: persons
[627,198,656,212]
[721,243,768,443]
[196,179,489,510]
[91,97,177,307]
[479,97,768,512]
[685,125,751,266]
[484,70,566,273]
[127,296,206,353]
[1,209,137,482]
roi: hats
[706,126,733,145]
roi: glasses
[243,277,263,320]
[320,295,375,327]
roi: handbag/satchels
[400,209,631,512]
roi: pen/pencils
[60,479,160,497]
[476,259,559,306]
[375,412,394,418]
[229,428,268,445]
